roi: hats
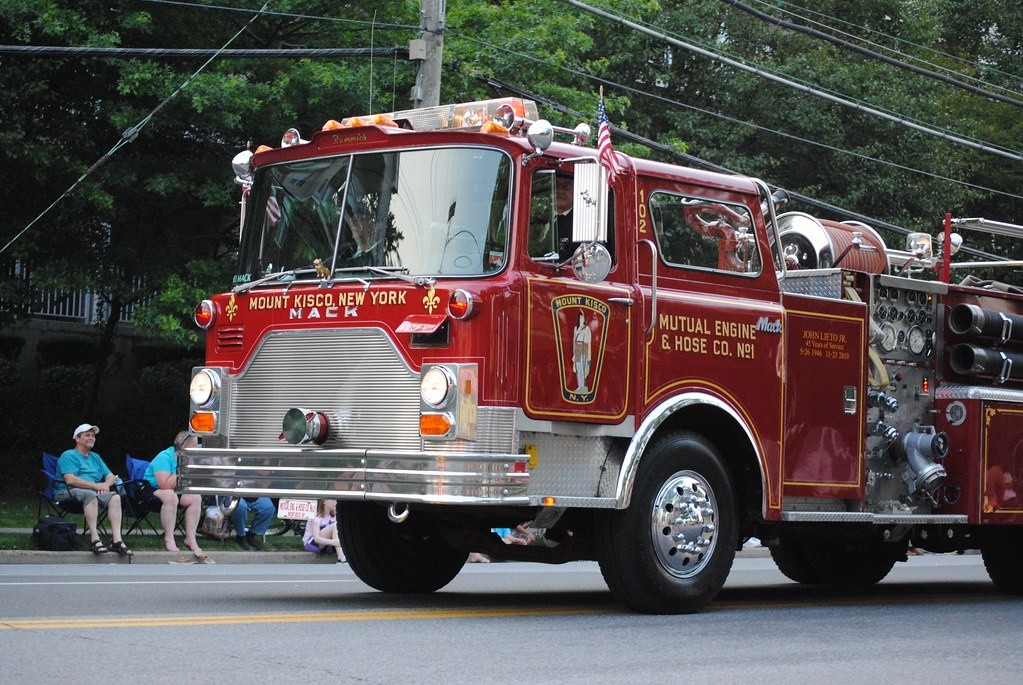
[72,424,100,438]
[174,430,192,448]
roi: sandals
[88,539,108,554]
[109,539,134,556]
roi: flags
[597,96,622,188]
[240,175,281,233]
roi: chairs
[120,454,187,547]
[37,452,130,551]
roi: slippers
[169,556,197,564]
[194,548,216,565]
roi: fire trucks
[167,92,1023,617]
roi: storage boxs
[199,509,234,538]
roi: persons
[145,431,347,566]
[56,423,131,555]
[524,171,586,265]
[338,206,391,265]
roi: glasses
[554,181,573,190]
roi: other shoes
[336,560,348,565]
[246,531,260,547]
[236,534,250,550]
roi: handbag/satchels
[116,477,163,512]
[31,516,78,550]
[200,494,231,539]
